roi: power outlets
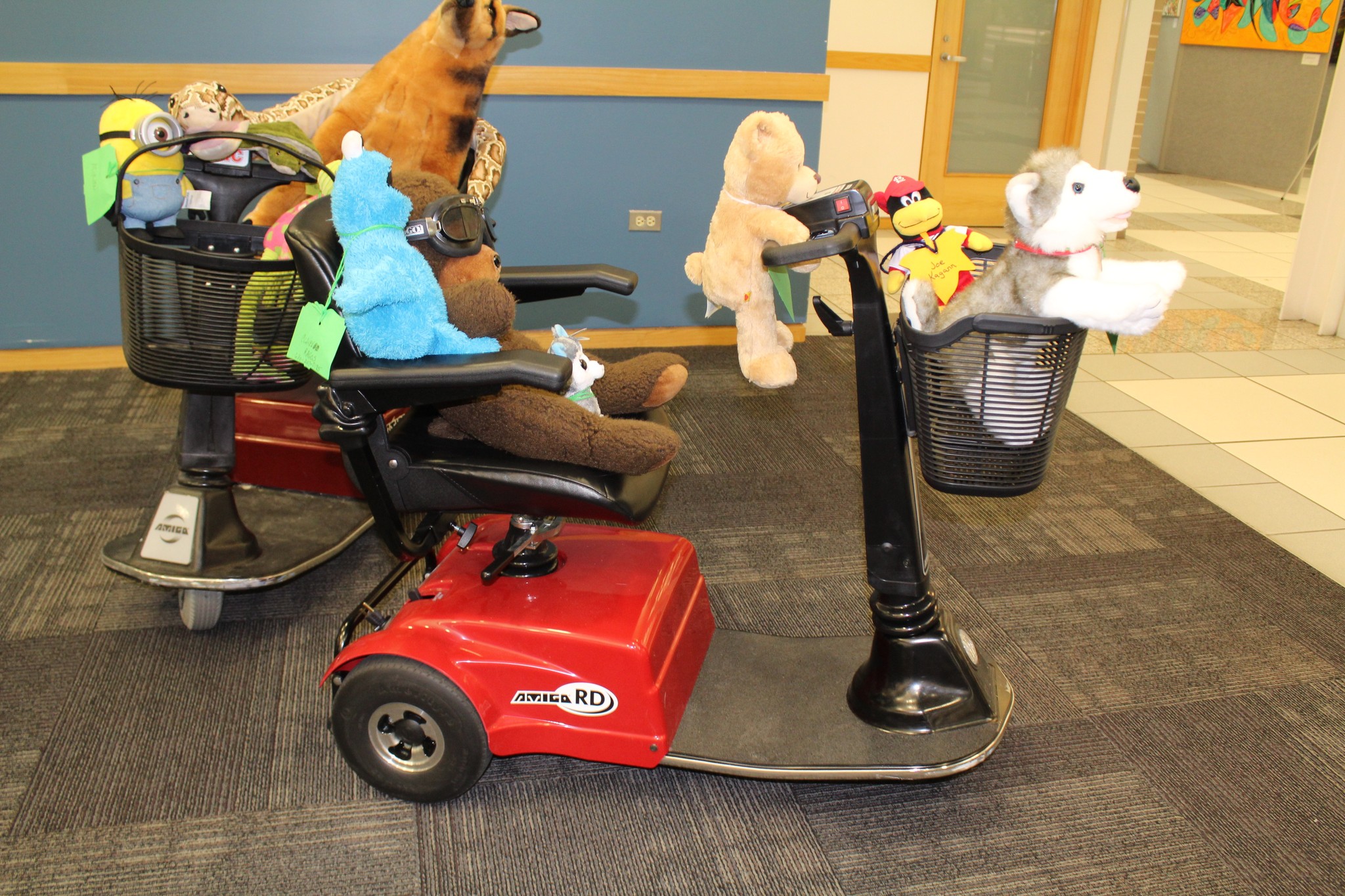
[628,209,663,232]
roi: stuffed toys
[899,145,1188,446]
[168,0,542,382]
[98,79,210,249]
[326,130,502,361]
[873,173,995,314]
[683,110,821,389]
[389,169,688,476]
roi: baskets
[899,244,1088,498]
[115,219,319,395]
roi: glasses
[408,193,498,256]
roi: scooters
[280,178,1091,809]
[98,124,408,634]
